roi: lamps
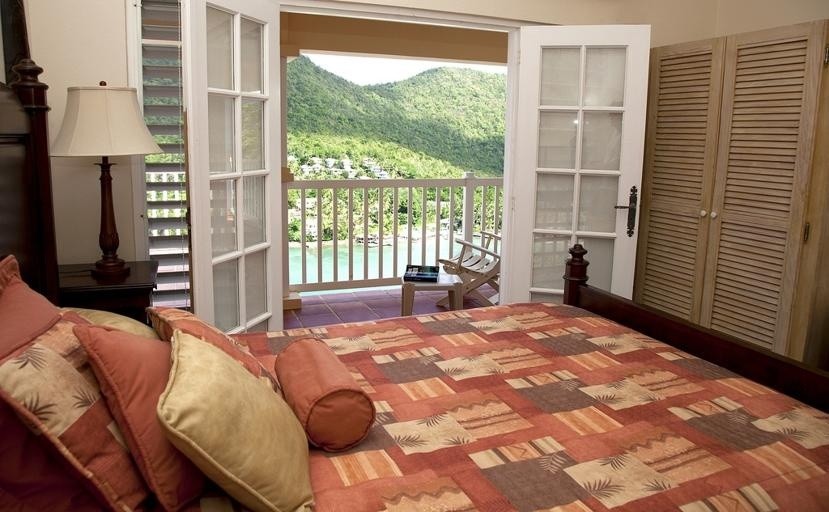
[47,81,166,278]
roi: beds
[0,57,829,512]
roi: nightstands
[48,260,161,328]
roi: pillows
[147,305,281,396]
[1,311,150,511]
[1,255,60,358]
[57,306,160,340]
[273,338,375,453]
[158,329,316,512]
[70,323,206,511]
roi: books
[404,265,440,282]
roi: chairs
[436,231,502,307]
